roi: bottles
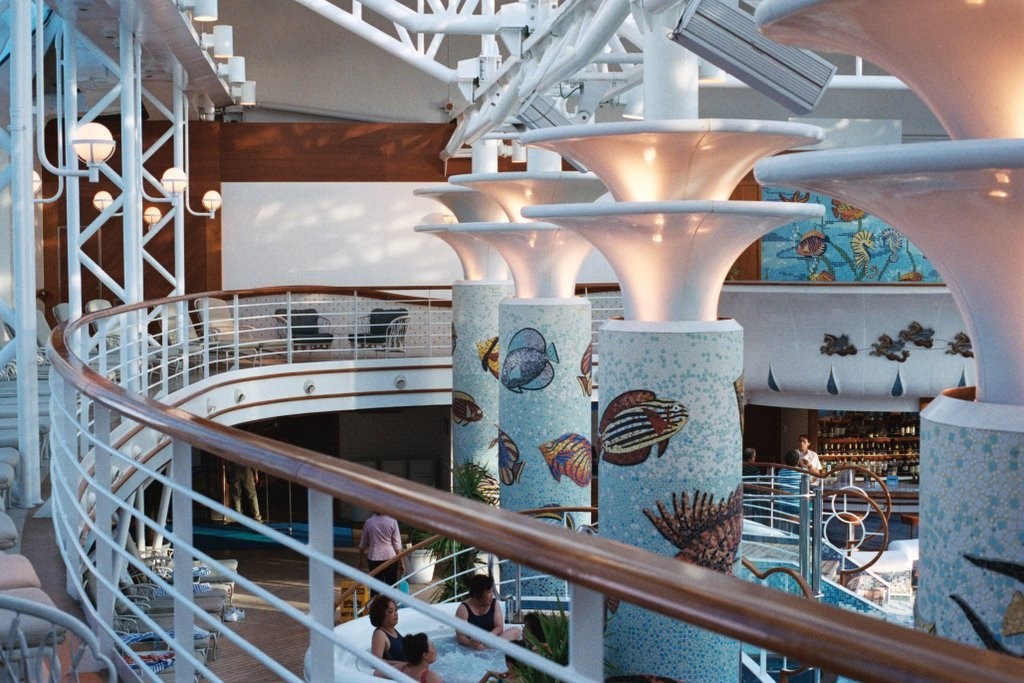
[819,458,920,483]
[816,412,920,438]
[819,441,919,455]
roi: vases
[402,548,437,583]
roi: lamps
[201,25,233,58]
[230,80,256,105]
[202,190,222,218]
[144,207,162,231]
[161,167,189,205]
[93,190,113,213]
[179,0,218,21]
[217,56,245,84]
[74,122,116,182]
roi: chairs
[0,296,410,683]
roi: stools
[901,513,919,539]
[840,511,863,552]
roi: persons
[776,450,802,533]
[796,433,822,490]
[742,447,761,522]
[222,457,263,523]
[454,575,520,651]
[479,640,533,683]
[374,632,443,683]
[522,611,553,655]
[358,513,406,614]
[369,594,406,664]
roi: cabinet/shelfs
[809,409,922,478]
